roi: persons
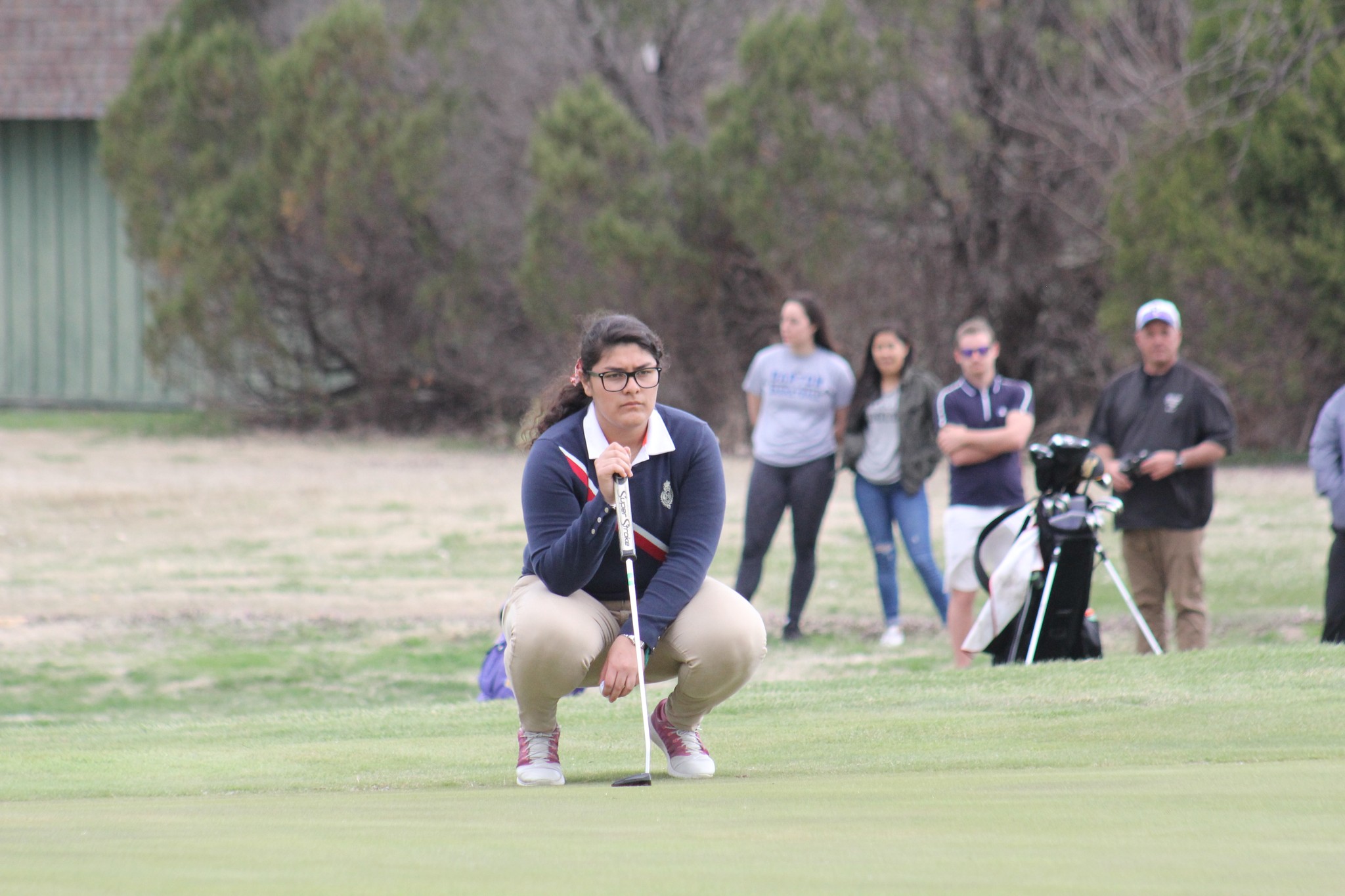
[1089,298,1234,655]
[936,315,1035,666]
[732,292,858,643]
[500,310,766,787]
[843,327,949,644]
[1309,382,1345,644]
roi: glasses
[586,364,662,392]
[959,341,997,357]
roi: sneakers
[649,697,714,780]
[515,726,564,786]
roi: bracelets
[1175,449,1181,472]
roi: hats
[1134,300,1181,329]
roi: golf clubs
[1028,433,1124,526]
[610,471,653,787]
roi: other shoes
[784,624,802,640]
[880,626,905,647]
